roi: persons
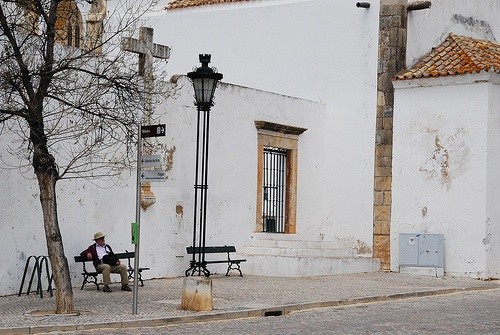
[80,232,132,292]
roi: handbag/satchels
[102,245,120,266]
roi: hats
[92,231,106,240]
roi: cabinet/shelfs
[398,233,445,277]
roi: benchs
[74,252,150,291]
[185,245,246,278]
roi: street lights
[185,53,224,277]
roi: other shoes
[121,285,132,291]
[103,286,112,292]
